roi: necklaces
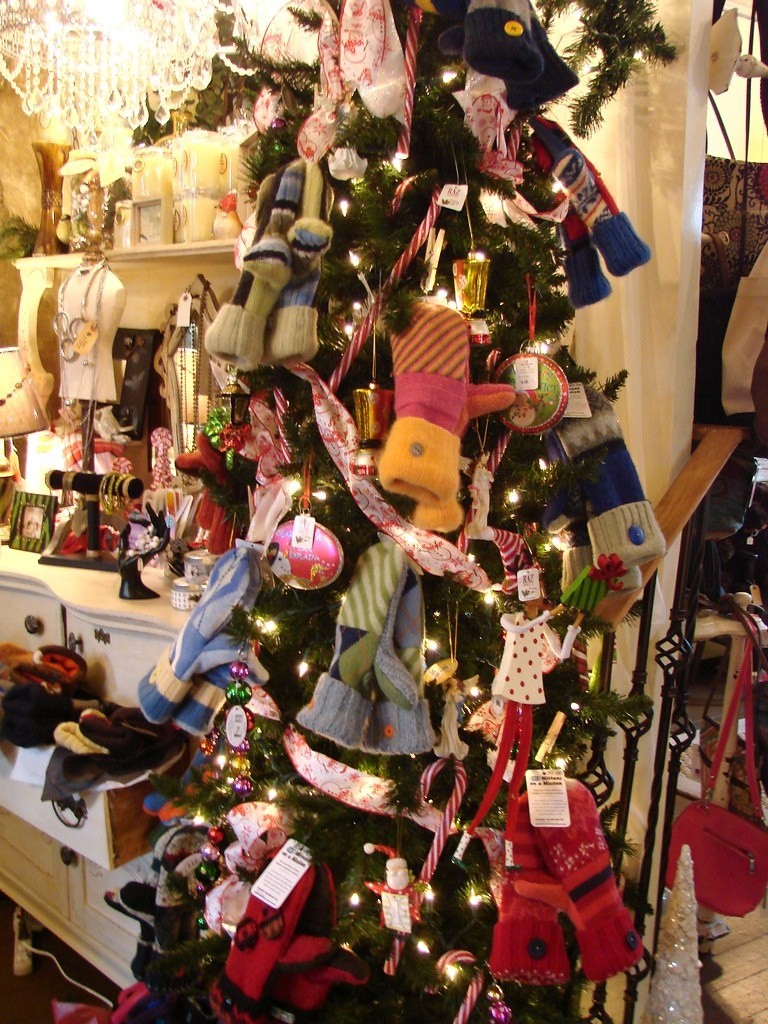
[54,262,109,464]
[0,361,31,409]
[173,282,211,485]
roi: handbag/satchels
[663,800,767,918]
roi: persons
[55,255,125,404]
[117,503,171,600]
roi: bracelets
[46,470,136,515]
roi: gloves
[175,555,264,735]
[537,431,643,594]
[548,189,615,309]
[489,786,572,986]
[103,824,225,991]
[263,859,371,1024]
[135,546,271,725]
[294,541,421,749]
[203,158,334,372]
[436,0,546,84]
[513,779,645,980]
[375,303,519,507]
[410,382,516,533]
[206,842,331,1024]
[555,386,667,571]
[529,114,652,278]
[495,1,580,110]
[360,564,437,758]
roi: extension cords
[13,906,32,976]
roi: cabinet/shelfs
[0,238,251,990]
